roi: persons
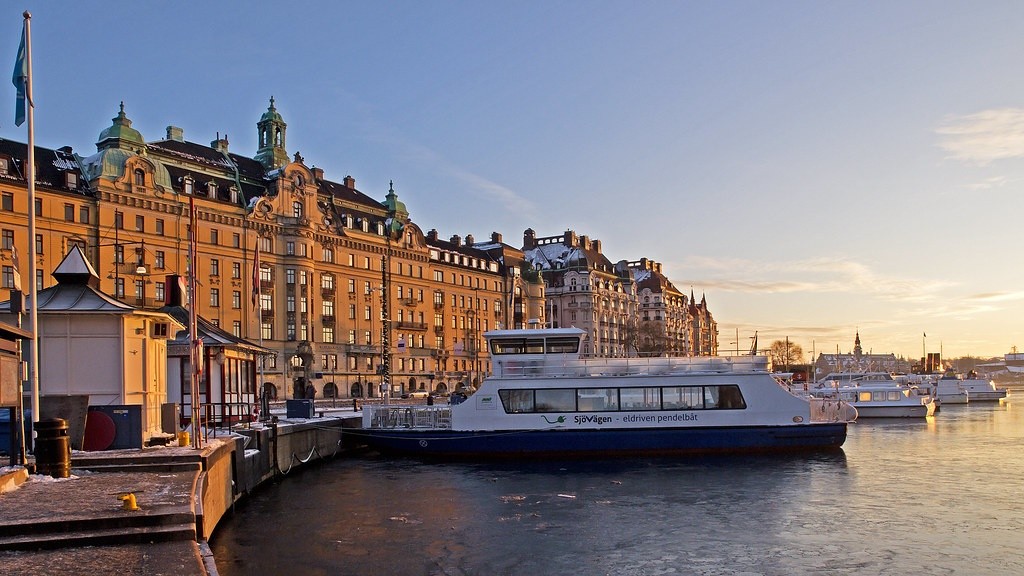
[425,393,434,406]
[304,381,317,416]
[446,393,451,405]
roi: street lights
[808,350,816,384]
[730,342,739,356]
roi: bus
[818,371,865,388]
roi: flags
[251,242,259,312]
[454,342,462,356]
[10,21,27,127]
[396,339,405,351]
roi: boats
[786,382,935,418]
[962,369,1011,400]
[813,374,941,412]
[892,332,969,404]
[340,317,849,456]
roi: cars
[459,385,476,394]
[400,389,430,398]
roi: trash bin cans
[163,403,180,441]
[32,416,72,478]
[0,408,25,456]
[286,399,313,418]
